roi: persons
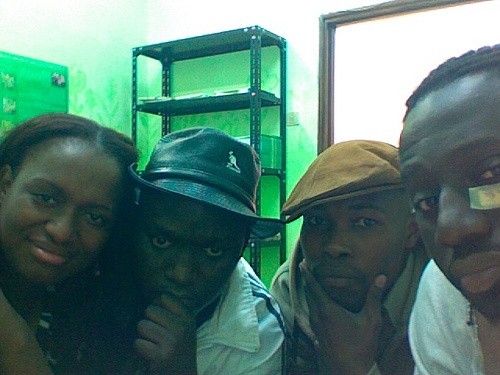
[0,115,283,374]
[271,140,431,375]
[399,44,500,375]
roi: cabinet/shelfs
[130,25,287,280]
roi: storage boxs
[235,134,281,170]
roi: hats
[280,140,405,224]
[127,127,286,240]
